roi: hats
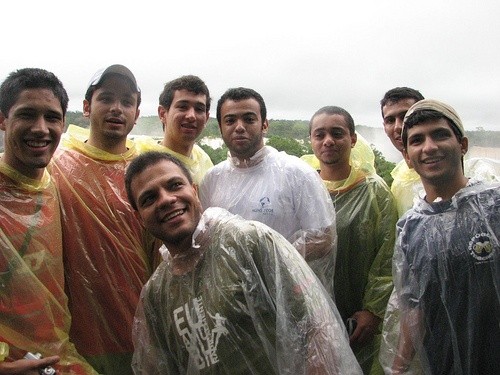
[401,98,465,145]
[87,64,141,92]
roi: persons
[378,98,500,375]
[195,88,337,305]
[125,151,363,375]
[300,106,403,375]
[380,86,426,220]
[50,64,164,375]
[0,67,100,375]
[136,75,215,186]
